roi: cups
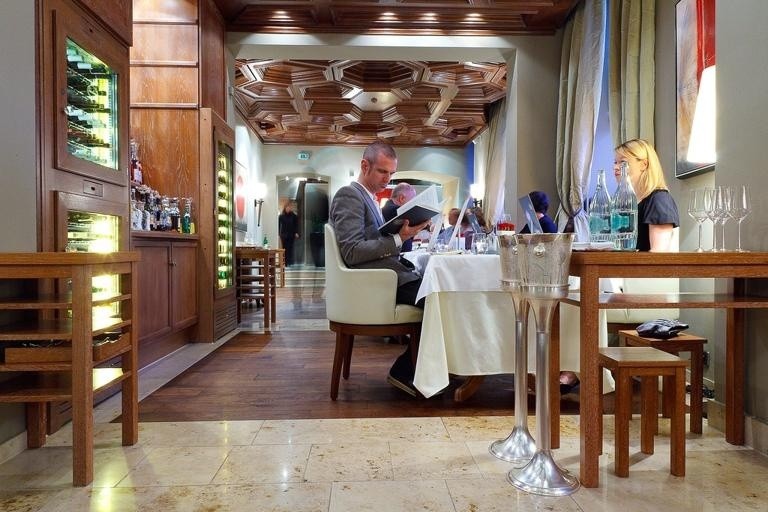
[427,231,497,256]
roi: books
[378,183,441,234]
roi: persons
[558,139,681,395]
[436,208,461,250]
[519,190,558,234]
[278,202,300,266]
[461,206,487,251]
[381,181,433,252]
[327,143,447,399]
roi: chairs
[324,222,423,400]
[607,226,681,414]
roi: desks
[1,250,139,487]
[272,249,286,288]
[236,249,277,328]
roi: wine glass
[684,184,752,253]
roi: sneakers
[386,357,445,399]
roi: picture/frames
[674,1,716,179]
[235,161,249,233]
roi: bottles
[611,161,639,250]
[263,237,268,248]
[129,138,193,235]
[216,152,234,288]
[67,212,94,250]
[65,57,115,163]
[588,169,612,242]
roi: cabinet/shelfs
[133,231,200,366]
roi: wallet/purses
[636,318,689,339]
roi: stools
[617,330,709,435]
[599,344,689,477]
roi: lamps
[470,183,483,207]
[254,182,267,227]
